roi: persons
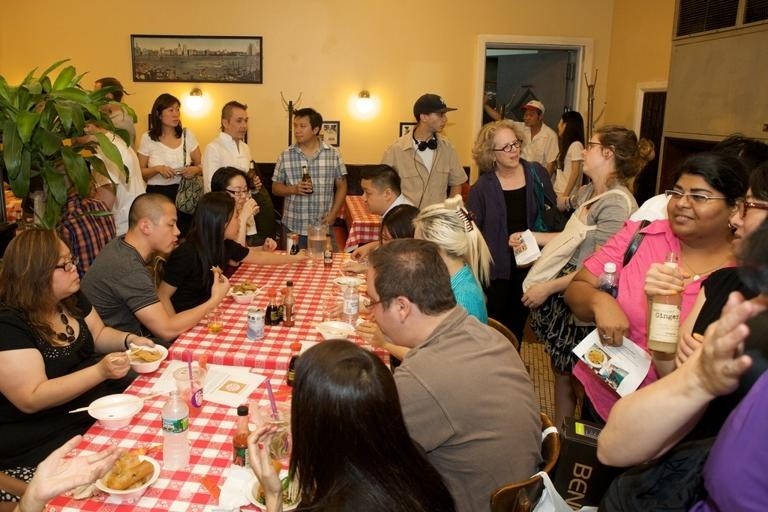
[208,165,278,254]
[365,238,543,511]
[0,225,156,504]
[379,204,417,244]
[359,164,412,217]
[270,107,349,253]
[137,92,201,237]
[157,191,311,318]
[202,99,262,247]
[80,192,230,341]
[412,194,493,325]
[0,76,145,273]
[380,92,468,204]
[13,434,123,512]
[245,337,458,512]
[467,94,768,512]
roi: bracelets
[124,333,132,351]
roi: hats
[413,94,458,116]
[520,100,546,115]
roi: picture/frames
[397,120,419,138]
[127,31,268,87]
[318,120,342,149]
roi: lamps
[344,87,381,117]
[183,85,218,119]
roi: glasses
[586,140,607,149]
[735,197,768,218]
[364,296,394,310]
[665,190,728,204]
[226,188,250,197]
[54,255,80,271]
[493,140,522,153]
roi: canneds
[246,307,266,341]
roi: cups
[207,308,225,334]
[262,404,291,460]
[307,223,329,260]
[172,366,206,420]
[240,201,259,216]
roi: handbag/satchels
[522,212,586,293]
[175,175,204,214]
[535,192,565,232]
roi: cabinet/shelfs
[654,1,767,197]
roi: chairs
[483,411,577,510]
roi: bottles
[246,161,257,192]
[300,165,314,194]
[161,390,190,472]
[285,341,302,386]
[264,280,296,328]
[644,251,684,355]
[596,261,619,294]
[324,233,333,269]
[230,406,252,468]
[342,281,359,316]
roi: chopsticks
[109,348,142,364]
[309,320,357,337]
[66,392,164,415]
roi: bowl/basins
[122,343,167,376]
[333,276,366,291]
[87,393,145,430]
[317,318,356,341]
[228,285,259,305]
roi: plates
[245,470,302,511]
[94,455,161,496]
